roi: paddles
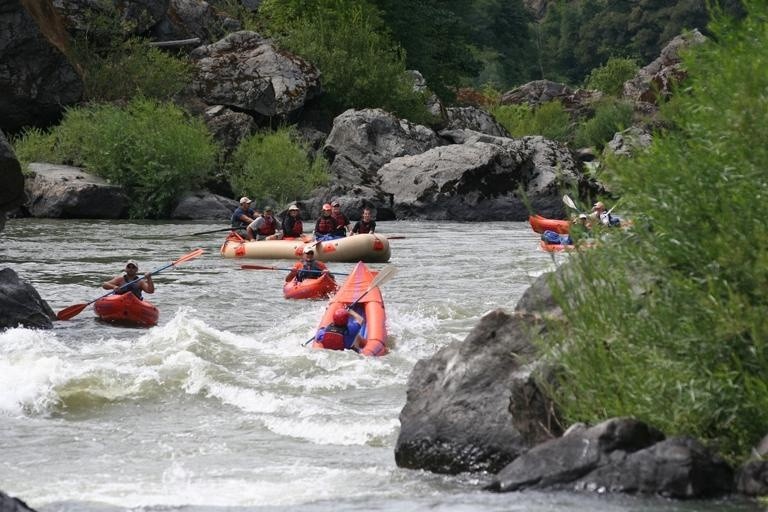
[562,194,600,233]
[302,265,398,347]
[241,264,379,277]
[311,233,328,248]
[57,248,203,321]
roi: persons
[588,201,611,228]
[314,302,367,354]
[331,201,353,237]
[282,204,305,241]
[285,246,329,282]
[102,259,155,301]
[246,206,283,241]
[231,197,260,243]
[568,214,588,245]
[352,207,377,235]
[314,203,343,241]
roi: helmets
[593,201,604,210]
[333,309,350,325]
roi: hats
[304,247,313,253]
[579,214,586,219]
[127,260,138,268]
[240,196,340,212]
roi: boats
[309,262,387,359]
[284,261,339,300]
[526,208,657,261]
[217,225,394,265]
[90,294,160,329]
